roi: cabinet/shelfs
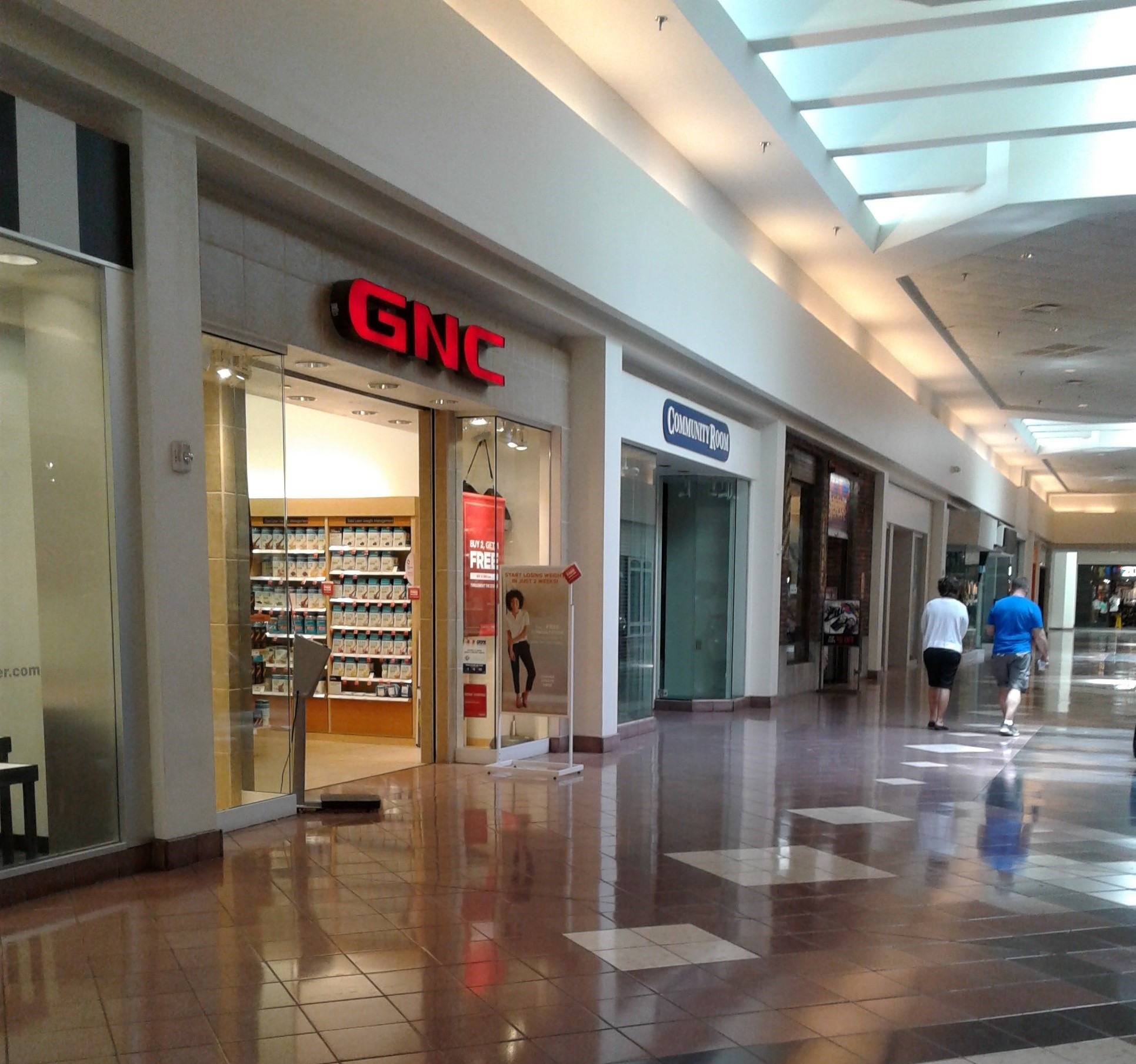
[250,496,421,739]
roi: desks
[0,763,39,866]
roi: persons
[911,735,958,889]
[842,603,859,623]
[987,577,1048,736]
[978,739,1046,898]
[1091,591,1120,627]
[507,589,537,708]
[921,575,969,729]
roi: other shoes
[928,721,949,731]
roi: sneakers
[999,722,1020,737]
